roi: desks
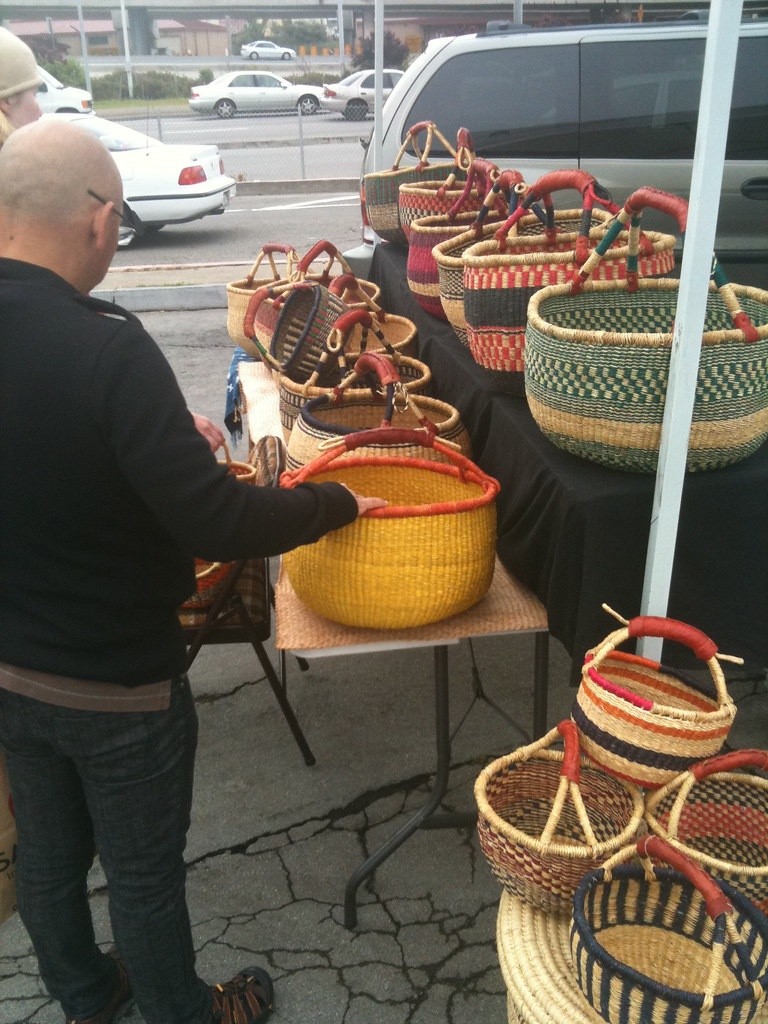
[238,359,555,938]
[364,240,768,690]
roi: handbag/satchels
[0,733,19,926]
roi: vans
[341,13,768,295]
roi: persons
[0,27,389,1023]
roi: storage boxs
[0,746,19,926]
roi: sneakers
[66,943,136,1024]
[201,966,275,1024]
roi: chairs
[161,434,321,771]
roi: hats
[0,26,43,98]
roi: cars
[319,68,404,122]
[189,71,325,119]
[240,40,297,61]
[36,66,93,114]
[40,112,238,247]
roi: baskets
[175,439,260,628]
[227,241,503,630]
[361,118,768,474]
[474,604,768,1024]
